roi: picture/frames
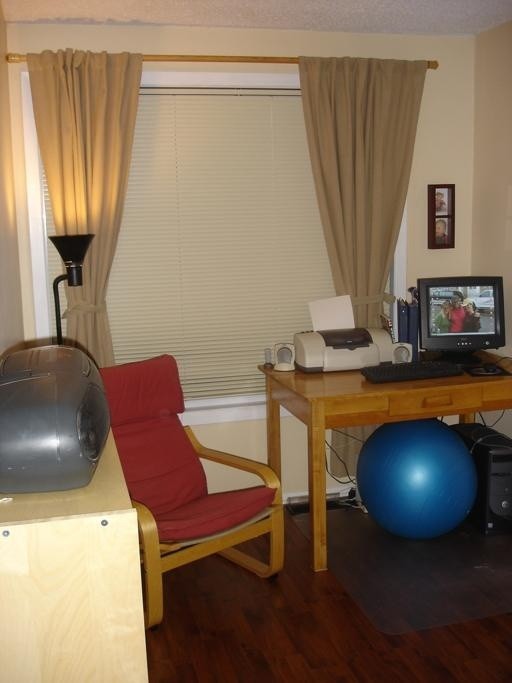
[427,184,455,249]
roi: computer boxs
[450,421,512,535]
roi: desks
[258,350,512,573]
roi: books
[380,294,419,362]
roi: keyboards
[362,356,467,384]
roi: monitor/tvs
[417,276,506,352]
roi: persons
[434,294,481,333]
[436,220,449,245]
[435,192,446,212]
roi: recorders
[0,344,111,494]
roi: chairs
[99,353,284,630]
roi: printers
[295,327,395,373]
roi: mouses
[484,362,496,372]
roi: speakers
[274,344,295,373]
[392,344,412,366]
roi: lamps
[49,234,95,343]
